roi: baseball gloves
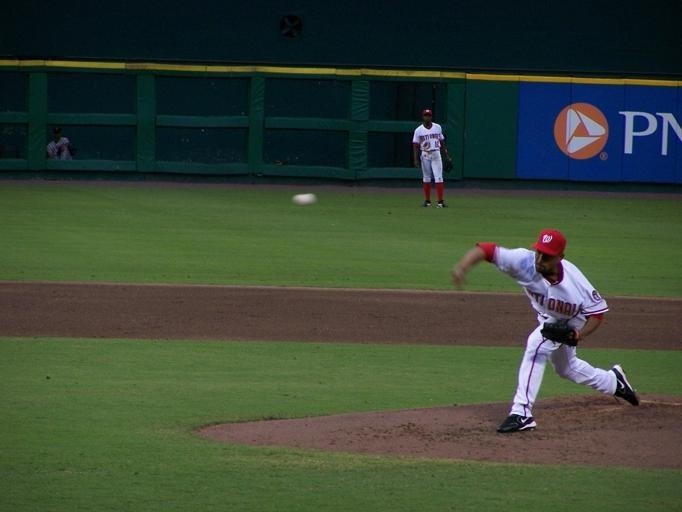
[444,158,454,172]
[541,319,579,347]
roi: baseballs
[293,193,315,206]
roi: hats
[532,229,566,256]
[422,110,432,115]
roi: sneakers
[497,415,536,432]
[425,200,444,208]
[612,364,639,407]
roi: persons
[46,125,77,161]
[450,227,640,434]
[412,107,454,209]
